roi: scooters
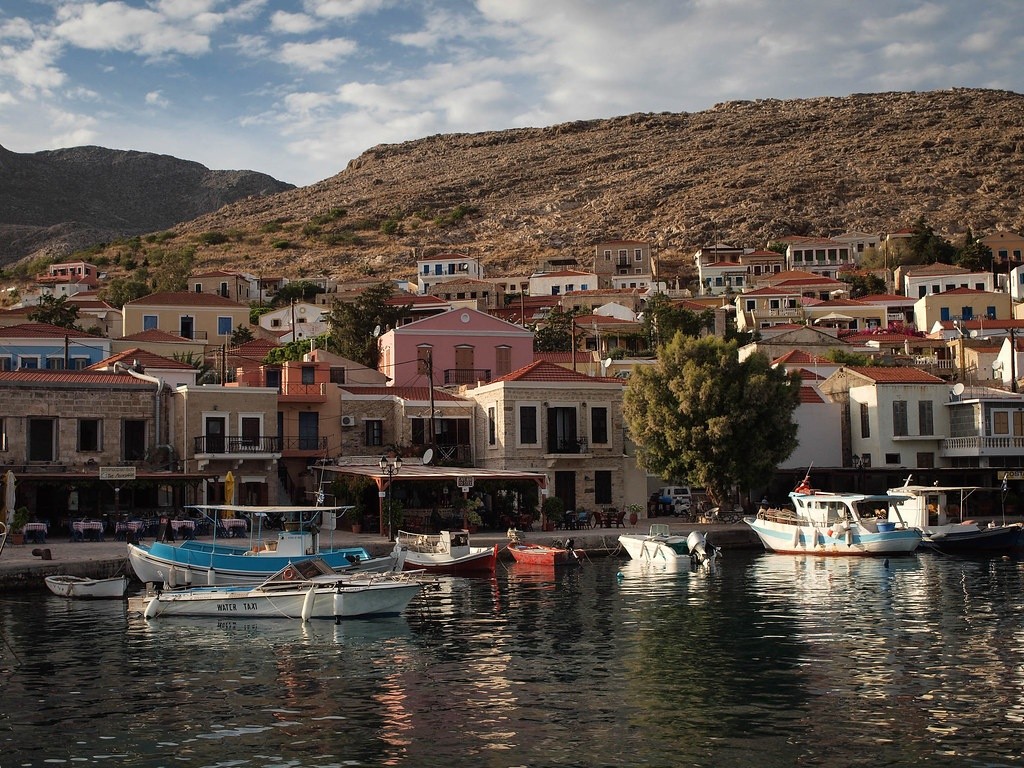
[673,497,692,519]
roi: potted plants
[382,498,403,537]
[9,506,29,545]
[540,495,563,532]
[464,497,483,534]
[626,503,644,525]
[345,504,369,533]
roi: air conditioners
[341,415,354,427]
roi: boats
[743,460,923,557]
[618,524,723,573]
[128,554,447,626]
[127,501,408,589]
[508,539,585,567]
[44,575,130,598]
[388,528,495,573]
[885,477,1024,558]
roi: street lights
[378,456,402,539]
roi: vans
[658,485,693,513]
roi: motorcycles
[263,513,287,530]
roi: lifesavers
[284,569,295,580]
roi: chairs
[556,508,592,531]
[704,507,720,525]
[610,512,626,529]
[20,517,251,544]
[493,510,537,532]
[592,511,607,528]
[608,508,617,521]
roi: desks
[116,520,144,539]
[602,512,616,527]
[20,521,48,543]
[221,518,247,536]
[72,522,104,540]
[159,518,196,539]
